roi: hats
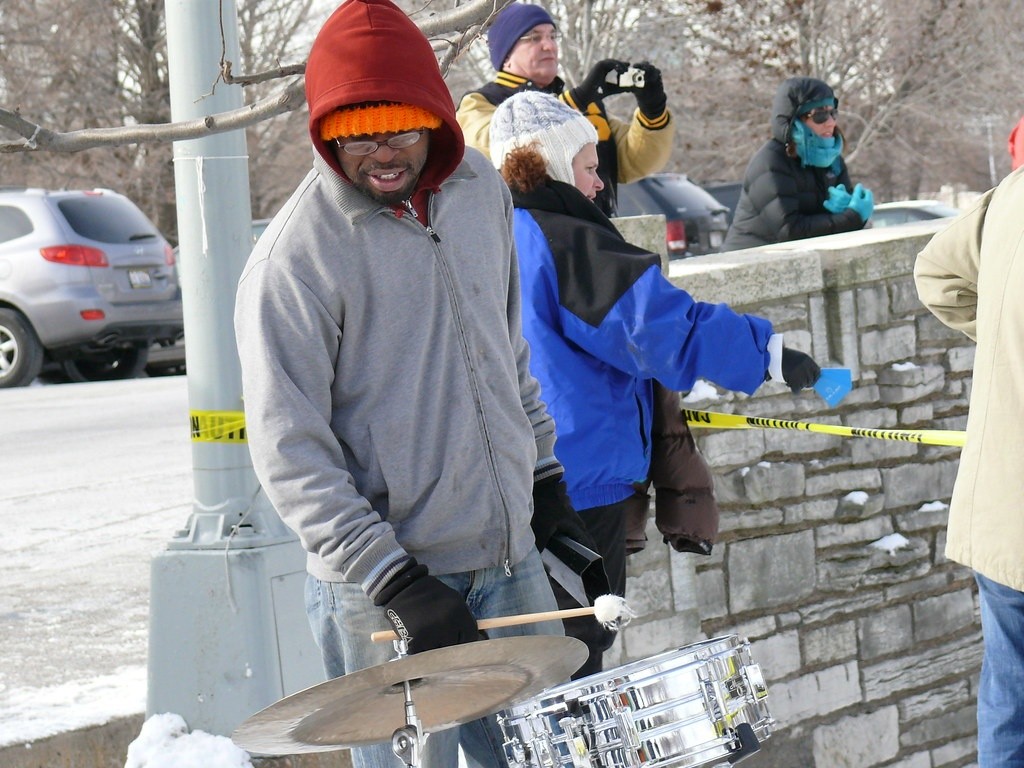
[489,1,557,72]
[489,91,598,186]
[319,101,442,142]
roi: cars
[865,199,963,228]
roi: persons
[914,165,1024,768]
[234,0,589,768]
[488,90,822,681]
[456,3,675,217]
[719,77,874,253]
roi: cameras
[618,66,645,88]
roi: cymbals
[230,634,590,755]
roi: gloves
[372,559,489,656]
[782,345,822,395]
[629,60,668,120]
[574,58,630,106]
[531,484,599,555]
[846,182,873,226]
[822,183,852,214]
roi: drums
[496,634,776,768]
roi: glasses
[333,126,425,156]
[802,109,838,125]
[520,30,564,48]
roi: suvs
[0,186,272,390]
[611,169,746,260]
